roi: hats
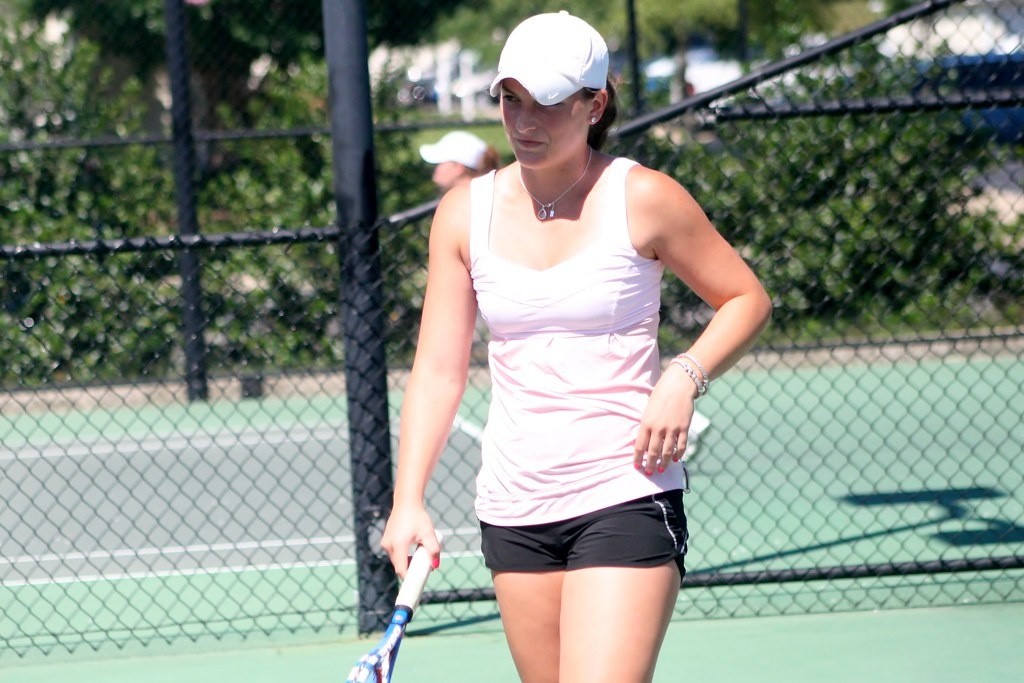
[490,10,609,104]
[420,131,487,169]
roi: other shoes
[681,410,710,462]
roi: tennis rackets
[345,528,445,683]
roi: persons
[381,9,773,682]
[418,130,711,464]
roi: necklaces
[514,143,593,220]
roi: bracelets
[670,352,711,399]
